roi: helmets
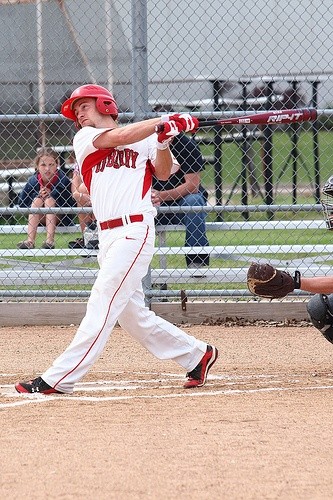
[62,85,118,130]
[320,174,333,230]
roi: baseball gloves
[246,262,295,300]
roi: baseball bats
[154,107,318,133]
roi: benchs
[0,220,333,285]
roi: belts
[100,215,143,230]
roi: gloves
[155,121,183,151]
[162,114,199,133]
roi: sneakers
[184,344,218,388]
[15,376,63,393]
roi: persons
[15,83,219,397]
[246,175,333,346]
[17,105,209,279]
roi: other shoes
[68,238,91,257]
[42,242,54,248]
[17,241,35,248]
[189,261,206,278]
